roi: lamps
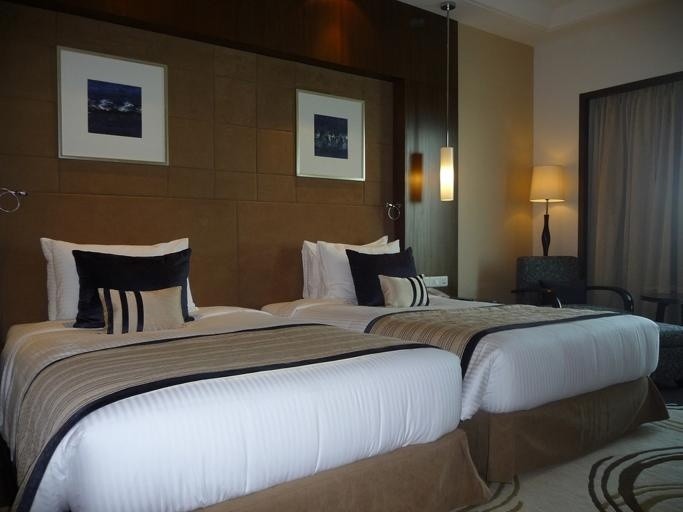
[439,1,455,203]
[528,166,567,256]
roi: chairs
[509,256,635,316]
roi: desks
[641,290,680,323]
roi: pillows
[36,235,200,337]
[300,234,430,308]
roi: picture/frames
[295,88,367,182]
[55,44,172,168]
